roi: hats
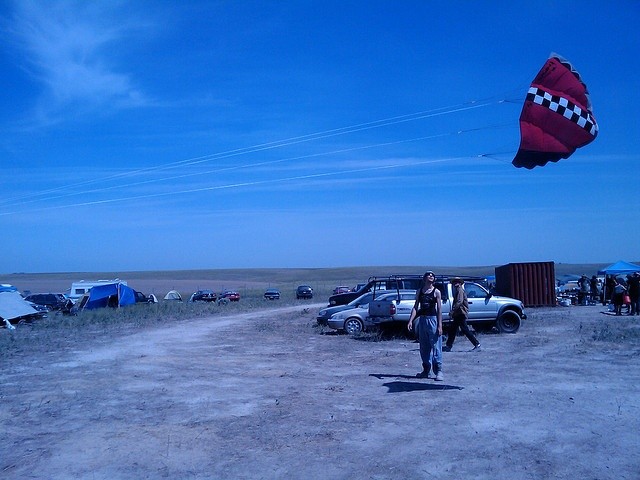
[450,277,464,284]
[423,270,435,281]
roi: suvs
[327,276,488,307]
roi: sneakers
[416,372,431,378]
[442,346,452,351]
[436,371,443,380]
[470,344,481,351]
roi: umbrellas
[597,260,640,275]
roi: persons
[590,275,599,300]
[408,270,445,381]
[605,272,640,316]
[443,275,481,352]
[578,274,591,304]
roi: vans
[24,293,62,312]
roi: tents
[164,290,184,301]
[149,293,159,304]
[77,283,138,309]
[0,284,47,329]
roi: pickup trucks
[369,274,527,333]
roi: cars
[199,289,217,302]
[333,286,350,295]
[316,290,390,326]
[264,287,281,300]
[296,284,314,299]
[352,284,371,292]
[328,293,416,333]
[219,290,240,301]
